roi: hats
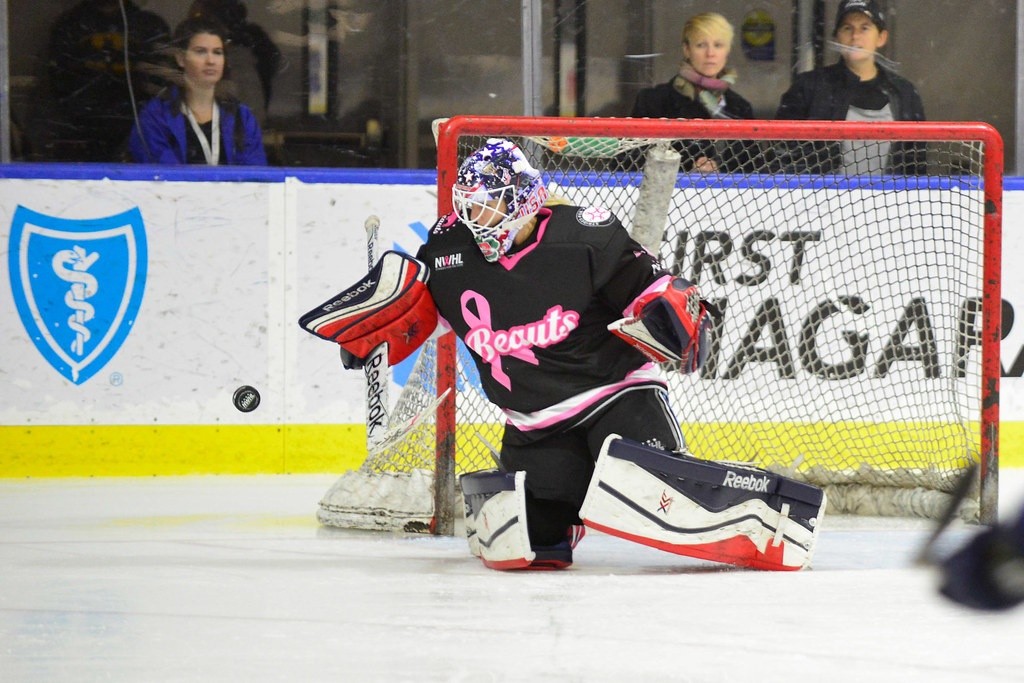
[832,0,886,36]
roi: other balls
[232,385,262,413]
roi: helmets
[452,138,548,262]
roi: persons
[130,24,267,167]
[174,0,281,112]
[28,0,171,163]
[613,12,763,173]
[775,0,928,176]
[299,137,828,571]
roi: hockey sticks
[353,203,441,459]
